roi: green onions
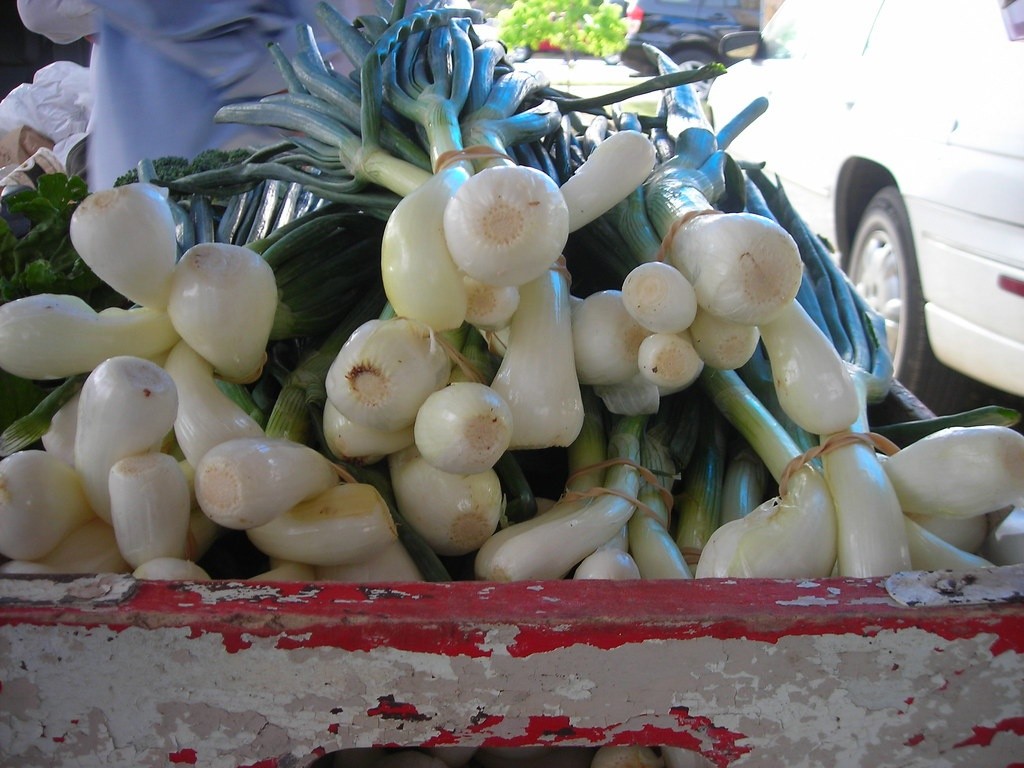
[0,0,1024,582]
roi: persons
[15,0,477,197]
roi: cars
[702,1,1024,407]
[622,0,762,83]
[506,14,620,67]
